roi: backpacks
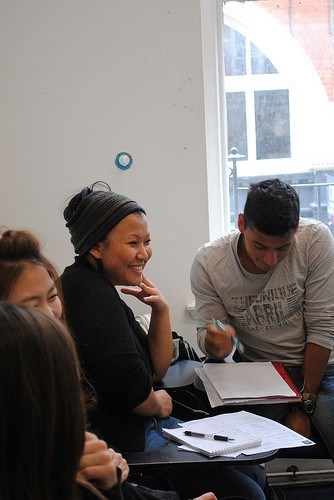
[153,331,229,421]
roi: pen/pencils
[211,317,249,357]
[182,428,237,446]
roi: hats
[66,190,146,257]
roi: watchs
[296,400,314,416]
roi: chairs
[154,359,202,389]
[123,441,280,467]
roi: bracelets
[301,393,316,402]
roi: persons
[54,181,268,500]
[191,179,334,464]
[0,229,217,500]
